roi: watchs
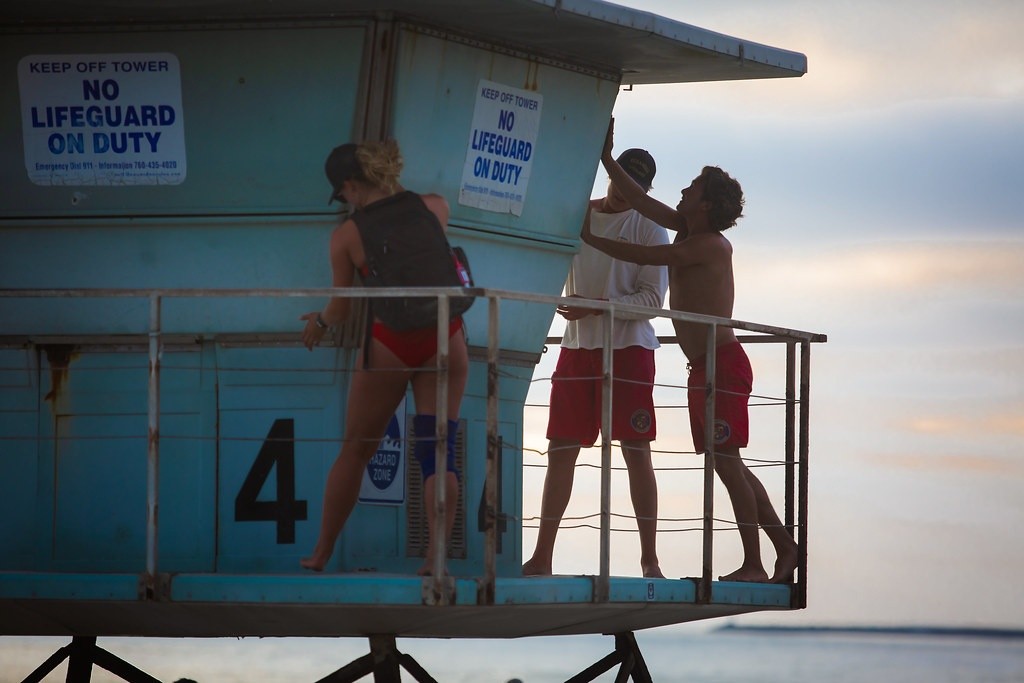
[315,312,329,329]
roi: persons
[298,141,468,575]
[580,116,800,584]
[522,148,670,580]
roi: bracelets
[591,297,604,317]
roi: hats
[325,143,363,206]
[616,148,656,188]
